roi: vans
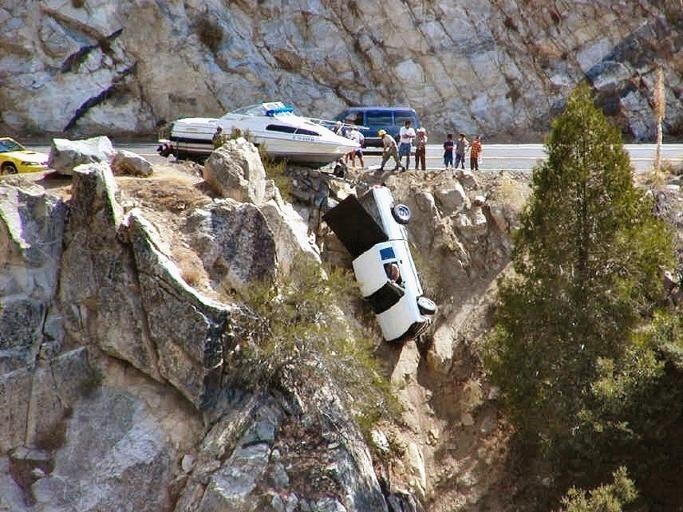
[323,102,423,149]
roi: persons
[468,136,482,170]
[329,121,365,169]
[413,127,428,170]
[392,121,416,171]
[213,127,223,142]
[376,130,405,171]
[454,133,468,169]
[386,263,402,285]
[444,134,454,168]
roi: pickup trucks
[338,183,439,346]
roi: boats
[156,100,371,171]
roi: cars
[0,136,51,177]
